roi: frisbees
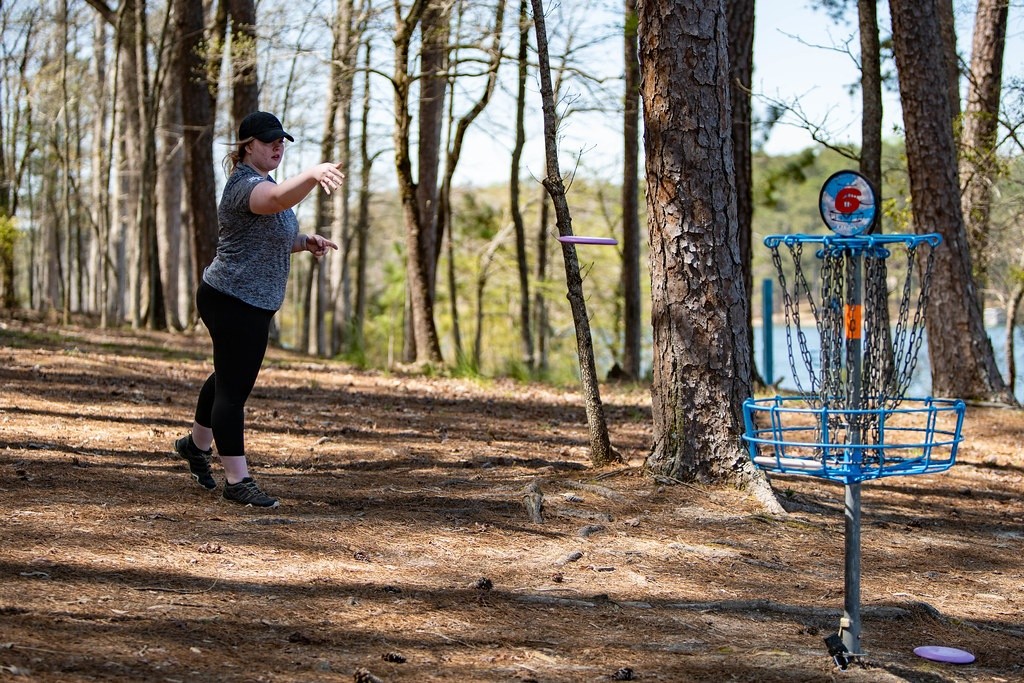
[913,643,977,666]
[557,235,620,246]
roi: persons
[171,111,346,512]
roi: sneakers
[222,477,279,509]
[174,429,217,491]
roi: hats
[239,111,294,144]
